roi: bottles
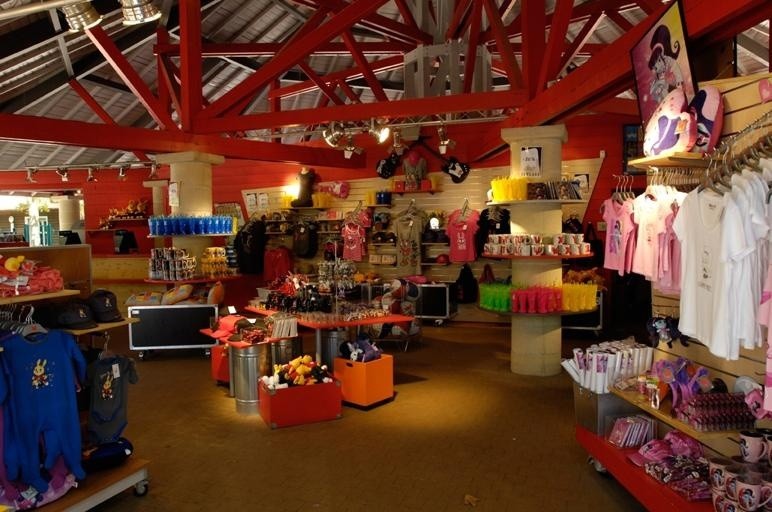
[147,213,235,239]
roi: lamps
[321,113,457,160]
[0,1,163,34]
[24,161,161,183]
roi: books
[608,413,656,450]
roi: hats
[60,290,125,330]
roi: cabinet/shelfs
[477,199,599,316]
[72,216,147,233]
[144,233,246,286]
[245,272,416,369]
[0,244,148,511]
[361,189,450,267]
[260,203,342,266]
[575,379,772,512]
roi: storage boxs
[258,371,342,430]
[212,344,230,385]
[332,353,394,411]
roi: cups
[148,246,194,279]
[708,427,772,512]
[483,233,591,257]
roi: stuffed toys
[262,331,383,390]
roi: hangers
[455,198,472,224]
[401,197,417,222]
[599,175,636,215]
[0,302,48,340]
[342,200,364,226]
[97,331,126,361]
[647,110,772,201]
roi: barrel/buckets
[271,334,304,377]
[319,331,350,373]
[232,342,271,414]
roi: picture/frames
[629,1,698,139]
[623,124,641,172]
